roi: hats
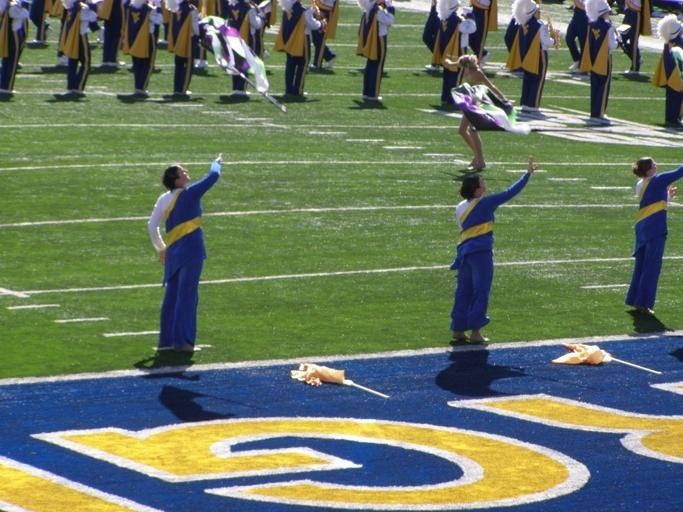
[585,0,611,21]
[512,0,539,25]
[658,14,681,43]
[436,0,458,20]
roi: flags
[289,361,352,388]
[550,342,611,365]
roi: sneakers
[454,332,488,342]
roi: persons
[622,156,683,316]
[147,152,223,352]
[448,154,538,344]
[442,52,514,175]
